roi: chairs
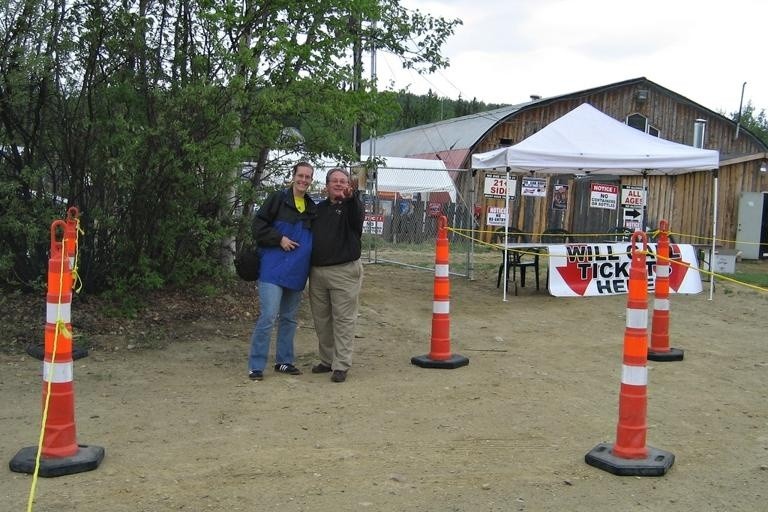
[604,226,675,244]
[492,226,578,297]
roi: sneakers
[312,364,331,372]
[331,369,347,381]
[249,370,263,380]
[275,362,300,375]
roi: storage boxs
[700,247,742,274]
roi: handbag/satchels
[233,237,259,281]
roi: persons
[309,167,364,382]
[248,161,318,380]
